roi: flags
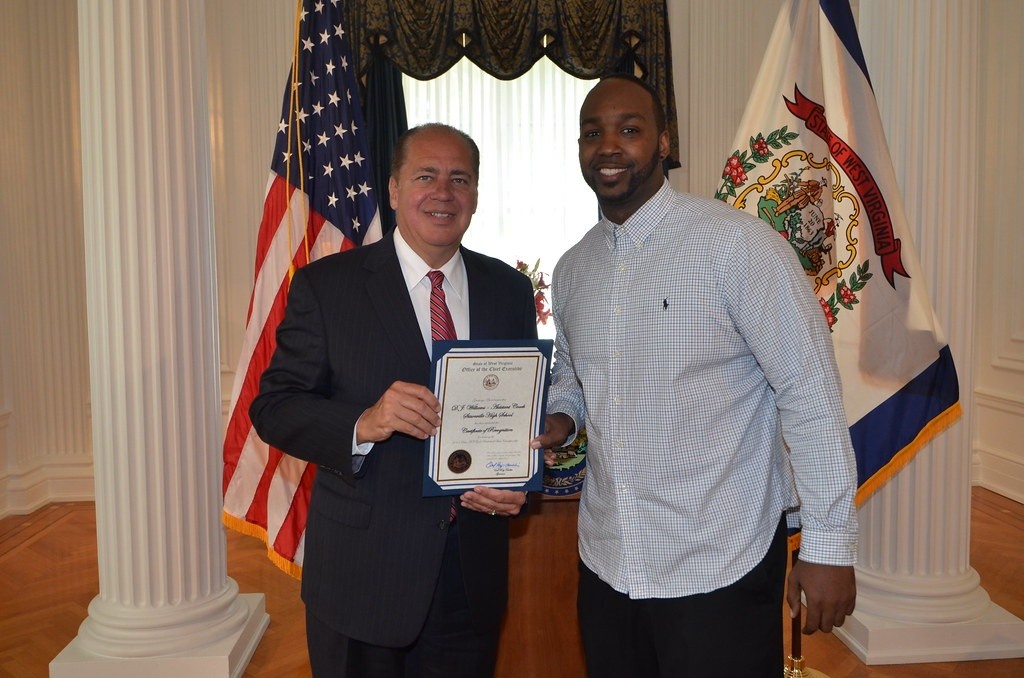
[711,0,963,552]
[222,0,384,582]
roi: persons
[526,74,858,678]
[248,122,539,678]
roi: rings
[489,509,496,515]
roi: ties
[426,270,459,522]
